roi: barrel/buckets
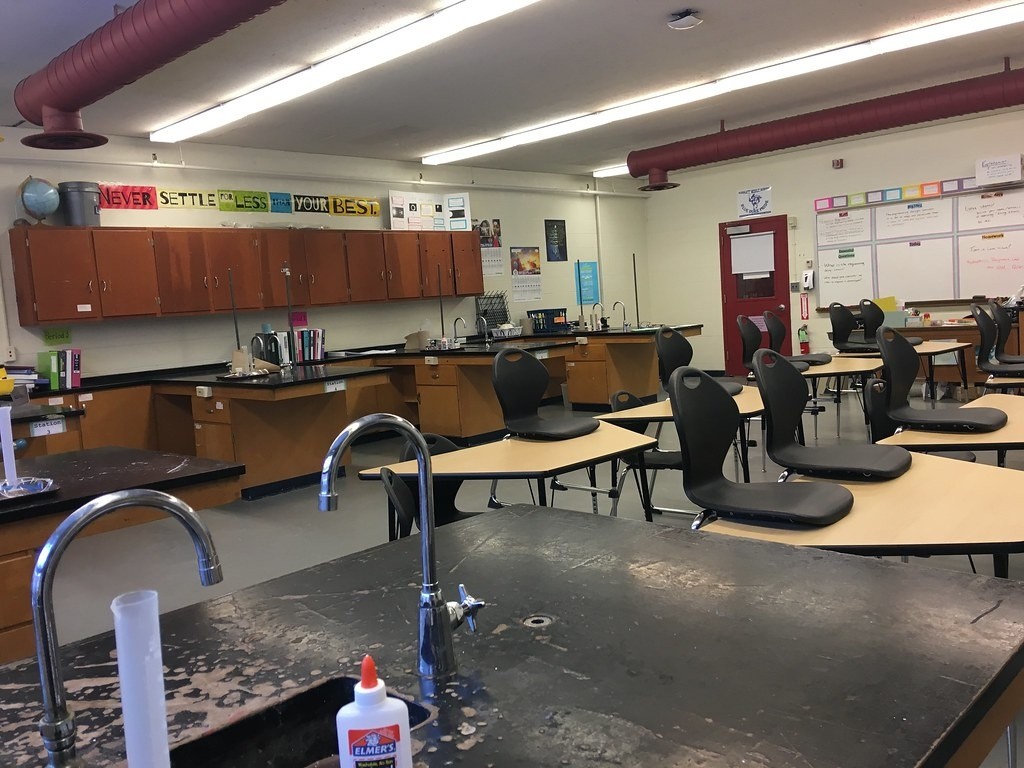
[58,181,101,226]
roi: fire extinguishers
[798,324,810,354]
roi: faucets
[592,302,604,318]
[454,316,467,343]
[475,316,493,346]
[30,486,226,768]
[267,335,293,370]
[612,301,631,330]
[250,335,264,372]
[317,412,487,678]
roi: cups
[262,323,271,332]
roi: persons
[471,218,502,247]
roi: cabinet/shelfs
[10,385,350,499]
[9,227,485,327]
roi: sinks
[167,673,432,768]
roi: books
[255,328,325,364]
[5,365,49,392]
[38,348,81,390]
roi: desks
[747,358,884,445]
[827,321,1018,401]
[985,376,1024,394]
[698,450,1024,579]
[875,393,1024,467]
[831,341,973,409]
[0,503,1024,768]
[359,420,658,541]
[593,385,765,500]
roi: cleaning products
[335,652,414,768]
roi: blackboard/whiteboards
[813,182,1024,314]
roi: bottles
[923,313,931,327]
[441,335,448,350]
[336,654,412,768]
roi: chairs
[380,298,1024,563]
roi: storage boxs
[527,308,568,333]
